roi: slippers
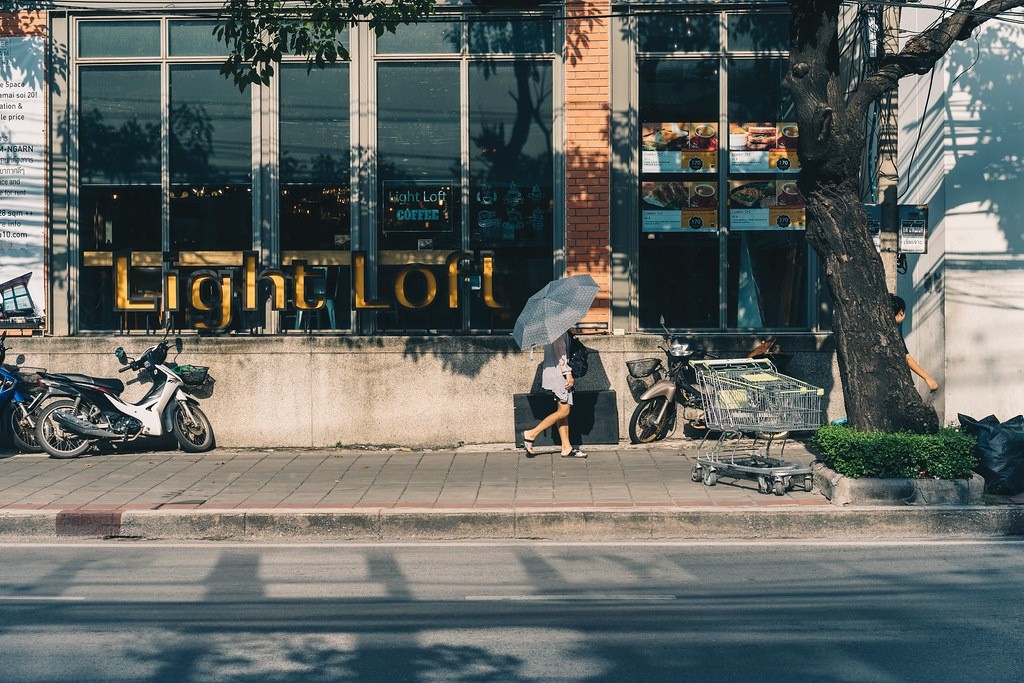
[561,448,588,458]
[521,431,534,455]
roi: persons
[521,330,588,459]
[891,294,938,393]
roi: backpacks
[567,330,589,378]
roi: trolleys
[692,359,825,496]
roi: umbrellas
[512,274,601,352]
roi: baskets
[181,364,210,386]
[625,358,662,378]
[12,366,47,387]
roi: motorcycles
[625,314,793,445]
[0,318,215,459]
